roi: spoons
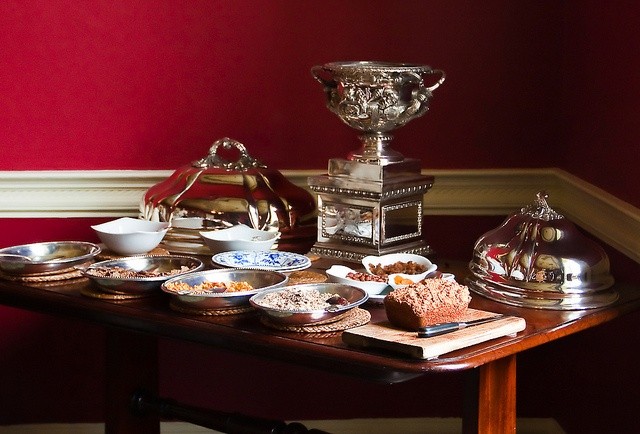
[326,296,349,304]
[0,254,65,265]
[74,261,161,275]
[178,285,227,296]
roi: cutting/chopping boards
[342,307,526,359]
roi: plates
[209,249,313,272]
[247,282,370,328]
[159,268,290,310]
[0,240,103,276]
[80,254,205,295]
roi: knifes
[417,313,523,340]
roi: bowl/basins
[361,253,439,292]
[90,216,171,257]
[198,222,282,254]
[324,263,395,304]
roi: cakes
[383,278,472,329]
